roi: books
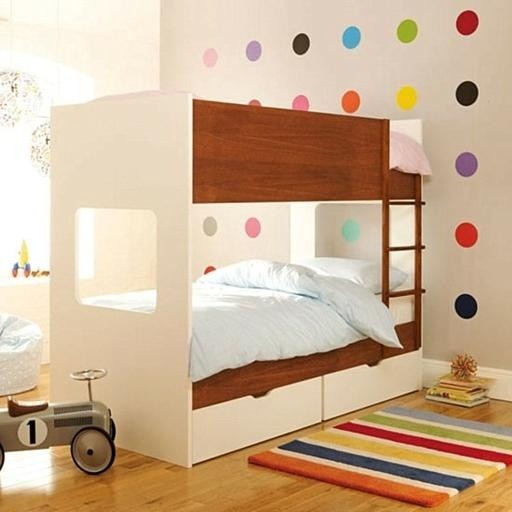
[424,372,498,409]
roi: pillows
[299,254,409,296]
[389,131,433,178]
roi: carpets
[247,403,512,507]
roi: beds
[46,91,422,463]
